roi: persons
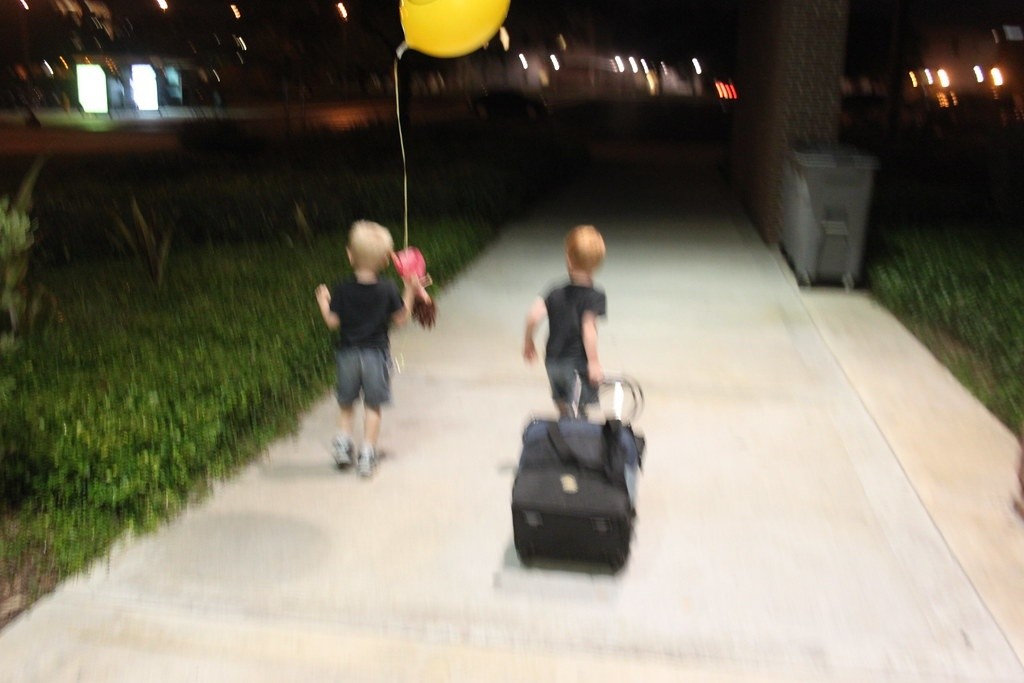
[523,226,608,421]
[315,221,416,479]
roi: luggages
[510,383,645,573]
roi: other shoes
[357,444,377,475]
[332,437,351,467]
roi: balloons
[399,0,511,59]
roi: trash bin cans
[782,140,879,289]
